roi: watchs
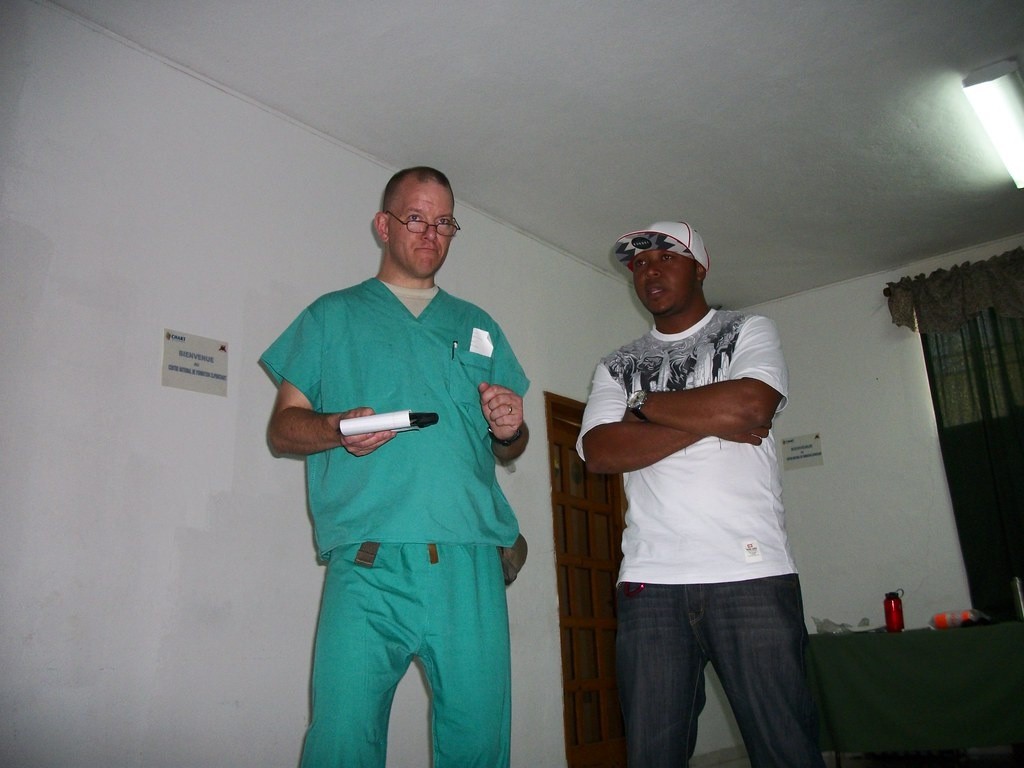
[627,390,652,422]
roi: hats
[614,220,709,272]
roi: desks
[807,620,1024,768]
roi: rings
[508,405,512,415]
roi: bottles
[884,589,904,632]
[1010,576,1024,622]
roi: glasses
[385,210,461,237]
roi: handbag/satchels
[495,533,528,584]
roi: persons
[575,222,827,768]
[260,167,532,767]
